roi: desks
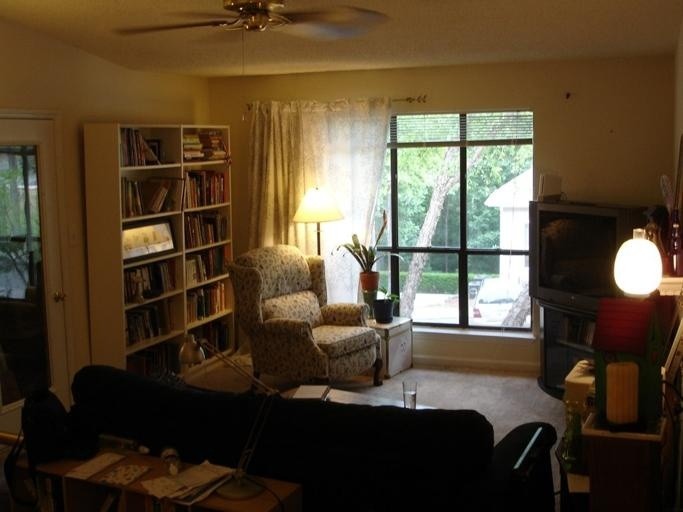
[14,447,302,511]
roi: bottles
[668,223,683,278]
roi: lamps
[179,334,280,500]
[614,228,663,298]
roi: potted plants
[371,285,401,323]
[330,209,403,319]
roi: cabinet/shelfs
[536,298,599,409]
[83,121,237,380]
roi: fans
[108,0,389,38]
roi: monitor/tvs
[528,200,649,314]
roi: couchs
[71,365,558,512]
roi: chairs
[226,244,383,394]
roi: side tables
[365,316,413,378]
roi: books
[122,126,229,372]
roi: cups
[401,380,416,409]
